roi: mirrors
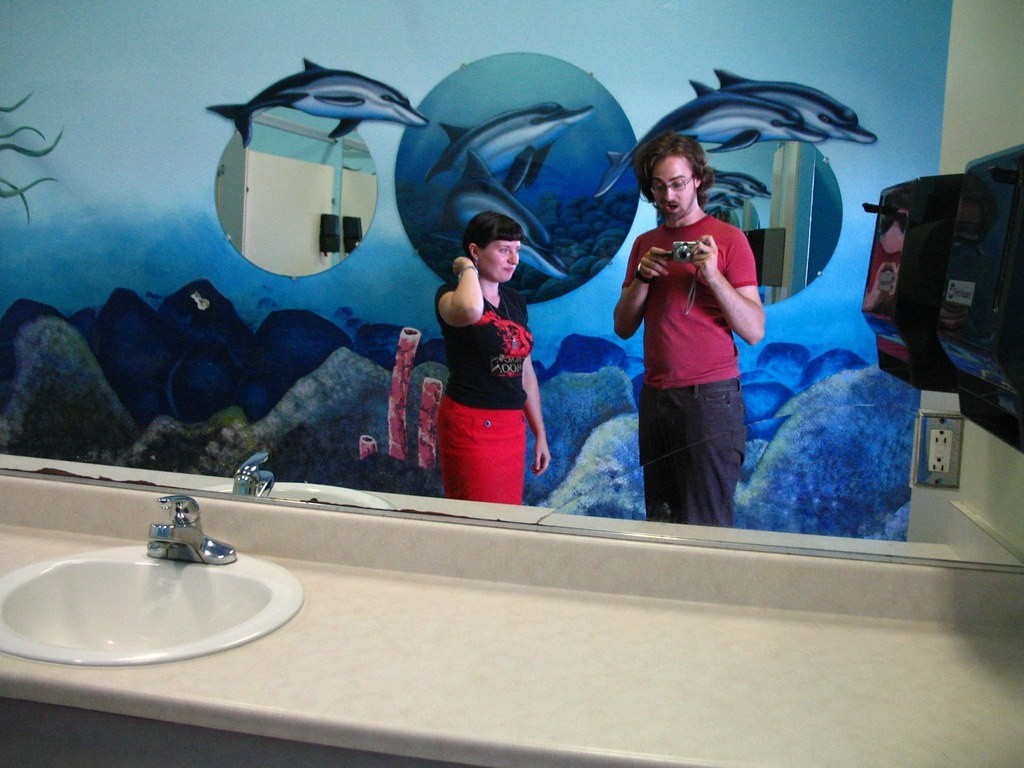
[0,0,1024,574]
[213,106,378,280]
[656,140,843,308]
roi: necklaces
[483,288,517,350]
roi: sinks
[203,480,393,514]
[0,539,305,667]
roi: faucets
[149,493,235,564]
[233,449,274,499]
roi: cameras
[673,241,705,263]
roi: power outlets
[909,408,965,493]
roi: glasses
[649,176,693,195]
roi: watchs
[634,262,653,284]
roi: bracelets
[458,265,478,282]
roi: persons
[613,130,765,528]
[436,210,551,504]
